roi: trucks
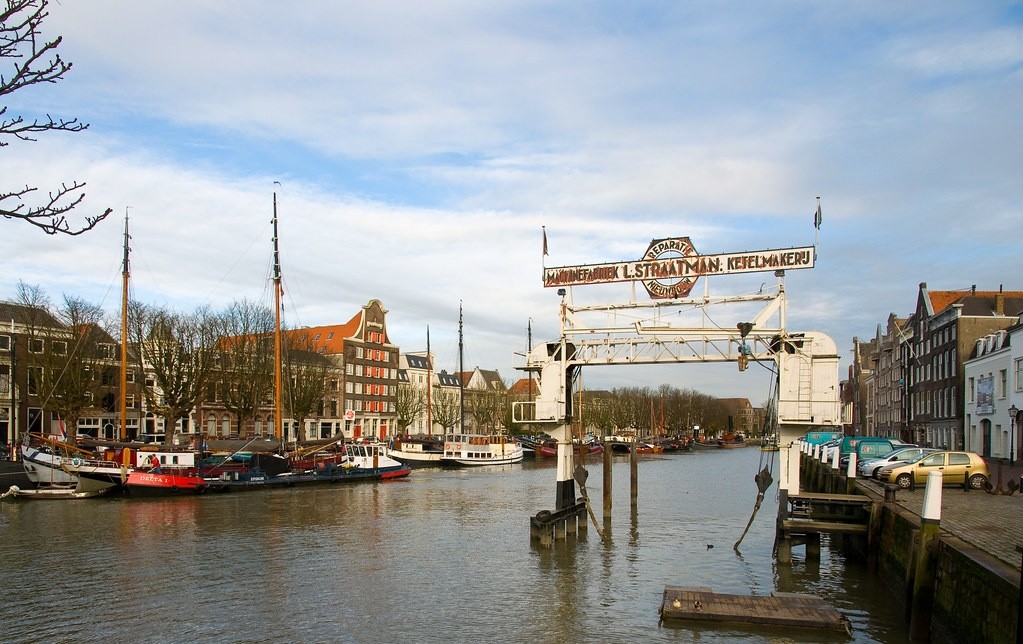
[798,432,844,450]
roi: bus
[131,432,179,446]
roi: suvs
[816,436,991,490]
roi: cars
[356,438,366,444]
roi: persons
[589,440,593,447]
[151,454,162,474]
[944,445,947,450]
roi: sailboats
[13,181,411,500]
[388,325,444,469]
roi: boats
[440,433,523,467]
[716,432,748,449]
[760,438,780,451]
[522,438,720,458]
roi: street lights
[1007,402,1018,466]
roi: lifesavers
[194,484,206,495]
[446,444,450,450]
[456,444,462,450]
[171,484,179,492]
[71,458,81,468]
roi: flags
[59,419,67,440]
[815,206,822,230]
[390,436,394,447]
[544,232,548,255]
